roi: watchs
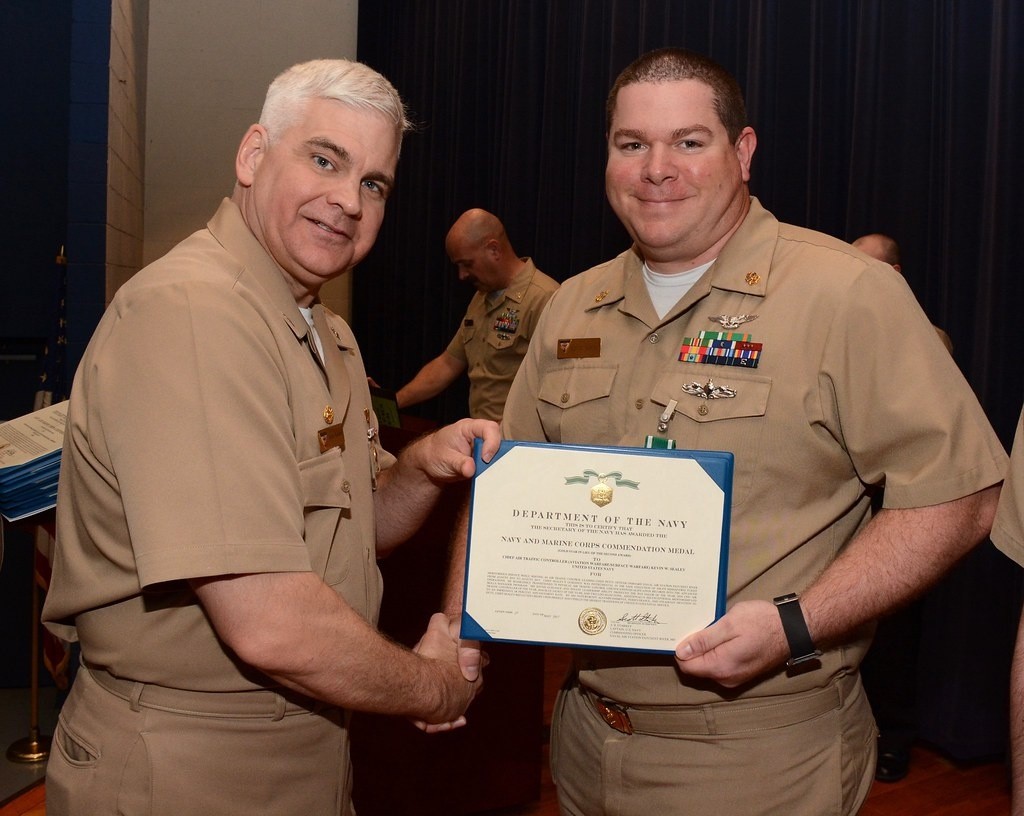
[773,593,823,667]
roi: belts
[86,663,336,717]
[583,673,858,736]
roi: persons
[367,207,560,427]
[990,405,1024,816]
[42,59,504,816]
[851,235,952,778]
[412,51,1010,816]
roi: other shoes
[876,743,910,783]
